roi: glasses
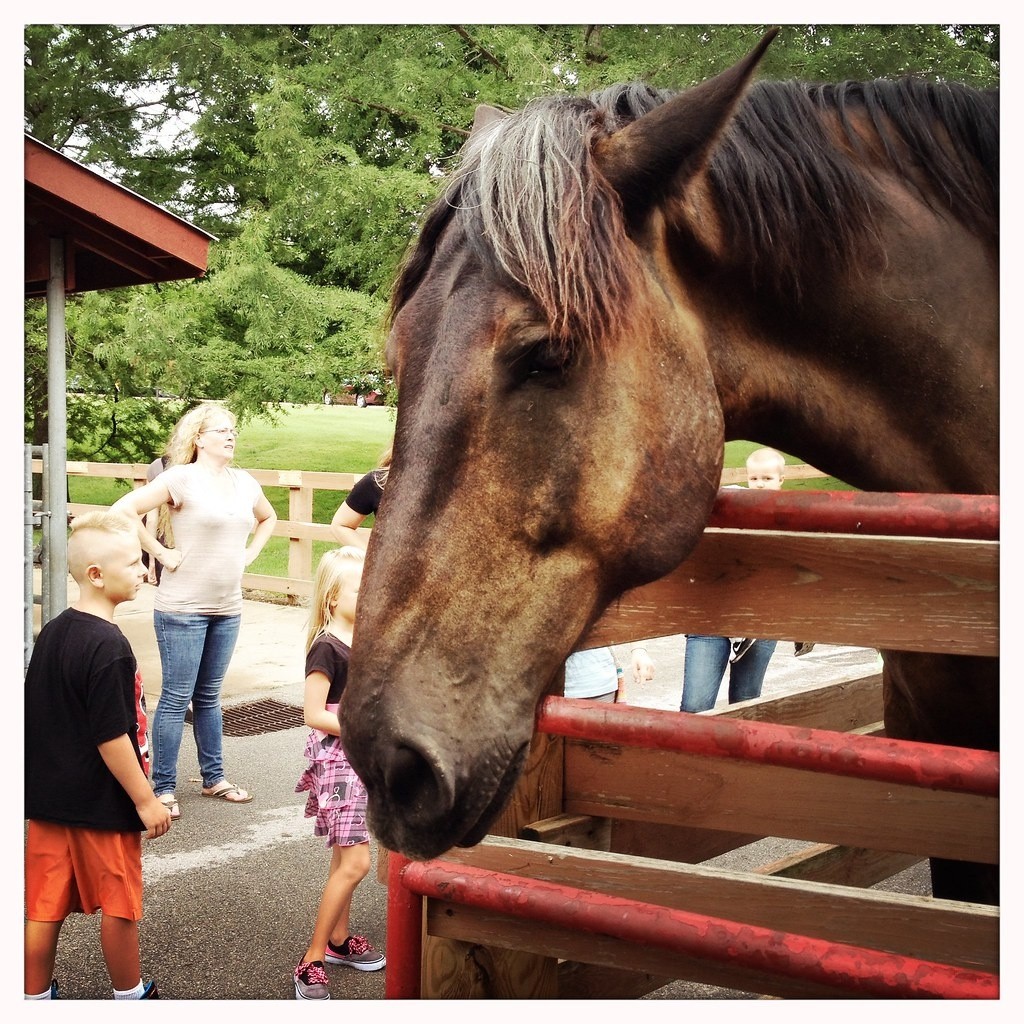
[199,427,240,439]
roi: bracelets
[155,547,167,559]
[631,647,647,653]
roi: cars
[323,370,392,407]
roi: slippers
[156,793,181,821]
[202,783,253,804]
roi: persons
[24,511,173,1000]
[111,403,277,818]
[293,545,386,1001]
[679,485,778,713]
[331,433,394,547]
[728,449,816,664]
[565,641,656,705]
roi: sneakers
[324,934,387,972]
[729,637,756,666]
[50,978,63,1000]
[794,639,814,657]
[138,979,161,1000]
[293,952,331,1000]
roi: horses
[337,24,1000,863]
